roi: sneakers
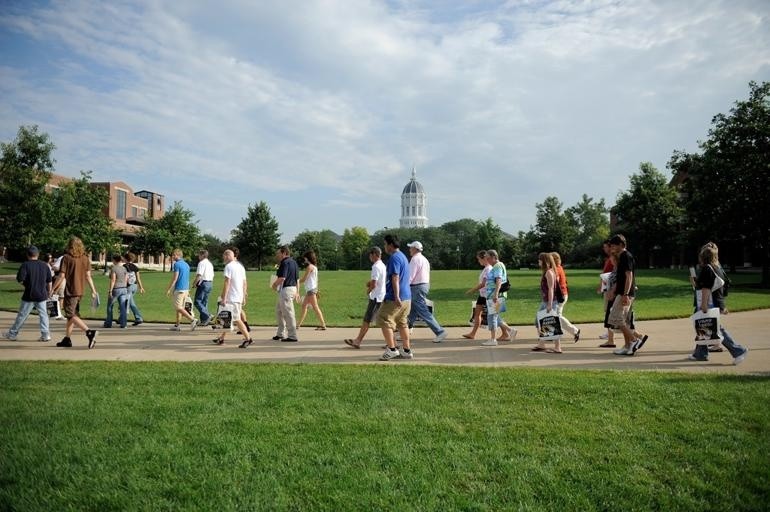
[498,328,517,342]
[273,324,328,343]
[688,354,708,361]
[599,332,616,348]
[344,339,361,348]
[480,340,498,346]
[103,316,143,329]
[85,328,98,349]
[633,335,648,351]
[0,332,18,340]
[614,347,633,356]
[37,334,52,341]
[432,331,448,343]
[379,328,413,361]
[709,346,723,352]
[169,318,255,349]
[56,337,73,348]
[463,334,474,339]
[733,347,749,366]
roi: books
[269,274,286,293]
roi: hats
[406,240,424,252]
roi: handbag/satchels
[535,309,564,342]
[44,292,67,322]
[600,272,613,293]
[467,299,492,329]
[721,276,734,297]
[183,296,195,318]
[415,298,434,322]
[498,282,511,293]
[712,275,725,293]
[687,307,727,346]
[124,294,131,314]
[211,300,236,334]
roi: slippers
[531,329,582,354]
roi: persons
[211,249,256,349]
[192,250,216,327]
[461,233,750,366]
[379,234,414,361]
[1,234,147,349]
[270,245,302,343]
[166,247,199,332]
[295,250,327,331]
[395,240,448,344]
[343,246,402,350]
[211,245,252,335]
